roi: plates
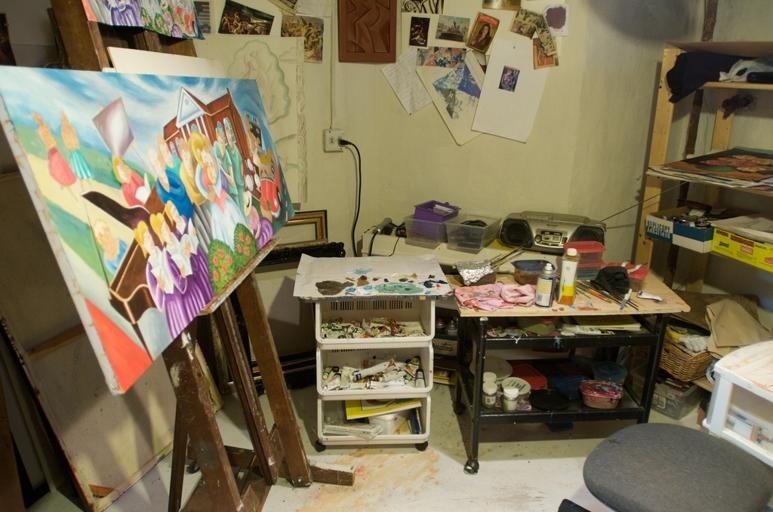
[468,356,513,381]
[499,376,531,396]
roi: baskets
[660,342,710,382]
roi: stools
[558,423,773,512]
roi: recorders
[499,210,606,256]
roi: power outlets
[324,129,346,151]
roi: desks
[360,225,595,386]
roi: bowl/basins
[511,259,554,284]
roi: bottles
[558,249,579,304]
[535,263,556,308]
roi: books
[344,399,423,421]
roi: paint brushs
[576,279,638,310]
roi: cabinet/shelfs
[634,47,773,392]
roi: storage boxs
[404,200,502,254]
[633,369,704,420]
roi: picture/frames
[208,209,328,397]
[229,242,345,395]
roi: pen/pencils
[620,288,632,310]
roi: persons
[500,68,517,91]
[410,19,467,67]
[470,23,492,50]
